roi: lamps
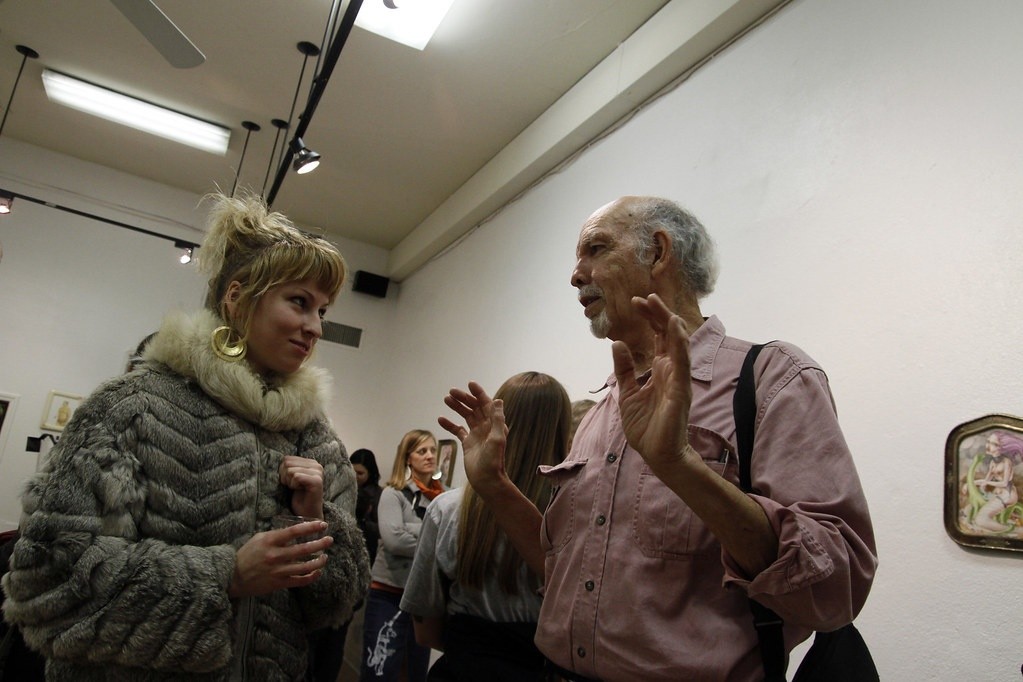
[0,192,13,214]
[175,241,194,265]
[289,136,321,174]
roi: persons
[398,371,572,682]
[347,447,382,569]
[362,429,452,682]
[437,196,879,682]
[0,180,374,682]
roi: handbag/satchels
[735,618,882,682]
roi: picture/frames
[0,392,21,462]
[944,413,1023,551]
[39,390,82,432]
[437,440,457,487]
